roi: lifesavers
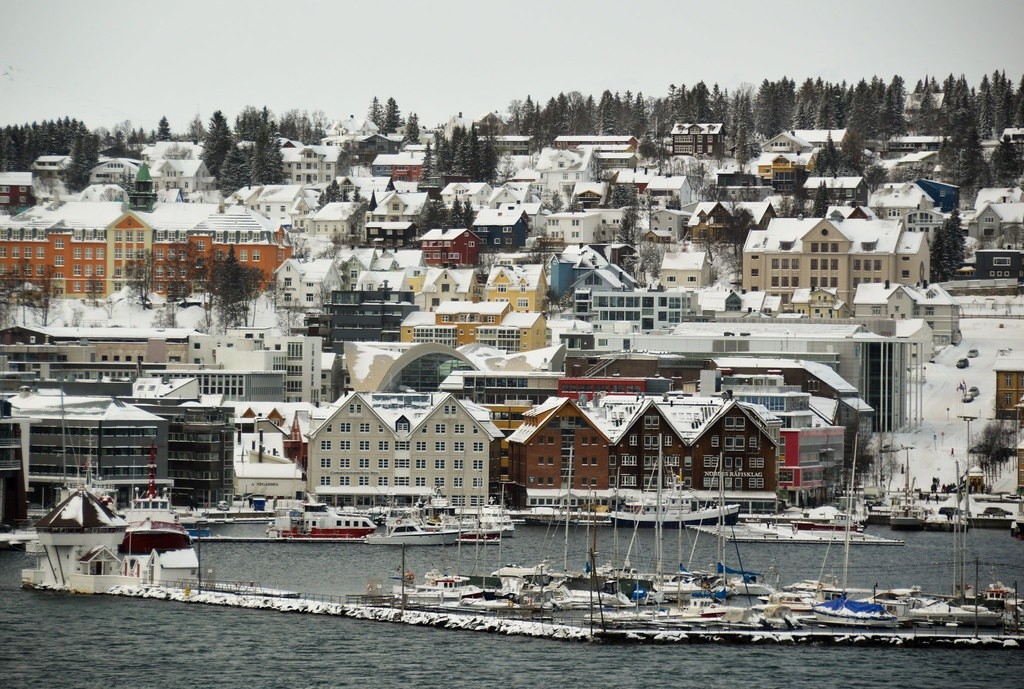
[818,583,822,589]
[508,601,513,607]
[642,508,646,512]
[396,520,401,525]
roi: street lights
[957,415,979,529]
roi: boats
[122,480,194,555]
[264,490,378,540]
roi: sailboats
[361,431,1024,642]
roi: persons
[935,494,939,504]
[925,492,930,504]
